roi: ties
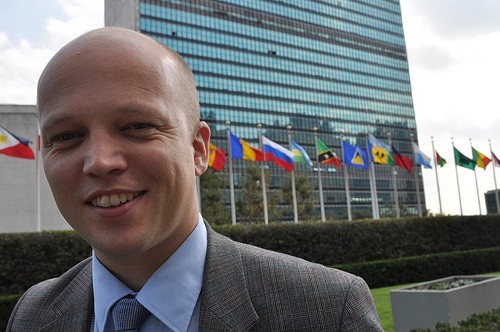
[110,294,151,332]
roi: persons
[6,26,385,332]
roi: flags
[435,150,446,167]
[0,125,34,160]
[491,151,500,168]
[261,134,294,172]
[472,147,492,171]
[413,141,432,169]
[454,146,476,171]
[317,138,340,173]
[291,140,313,166]
[230,131,263,162]
[208,143,226,171]
[392,143,413,173]
[343,140,369,170]
[368,132,394,167]
